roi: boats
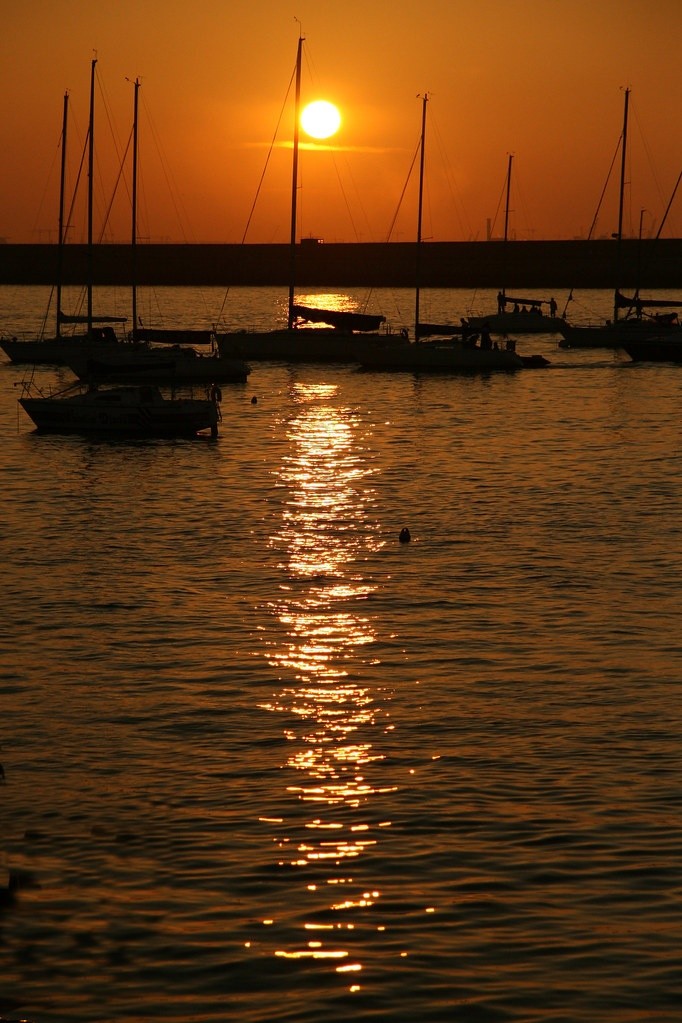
[416,323,467,336]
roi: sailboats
[2,34,410,385]
[347,88,542,374]
[13,75,229,440]
[558,87,682,366]
[462,155,573,333]
